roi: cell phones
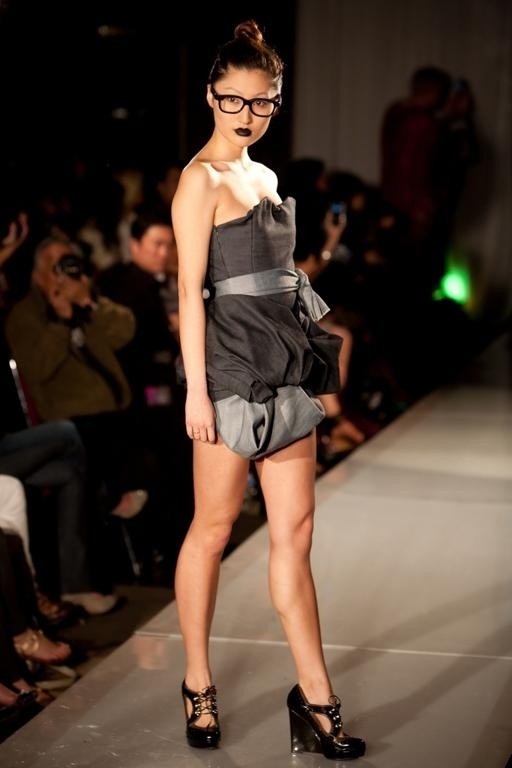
[329,201,342,227]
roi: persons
[379,65,445,221]
[431,80,494,247]
[171,18,373,761]
[1,151,421,739]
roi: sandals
[1,593,77,714]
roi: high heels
[181,679,221,749]
[285,682,367,761]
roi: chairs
[8,355,164,574]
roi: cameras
[53,255,84,282]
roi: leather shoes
[60,590,118,615]
[114,488,149,519]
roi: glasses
[211,86,284,119]
